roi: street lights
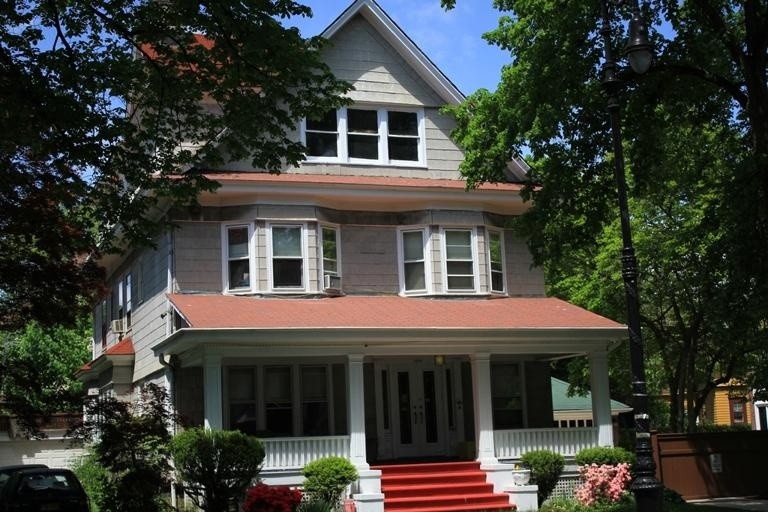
[601,2,661,512]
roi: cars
[0,463,90,511]
